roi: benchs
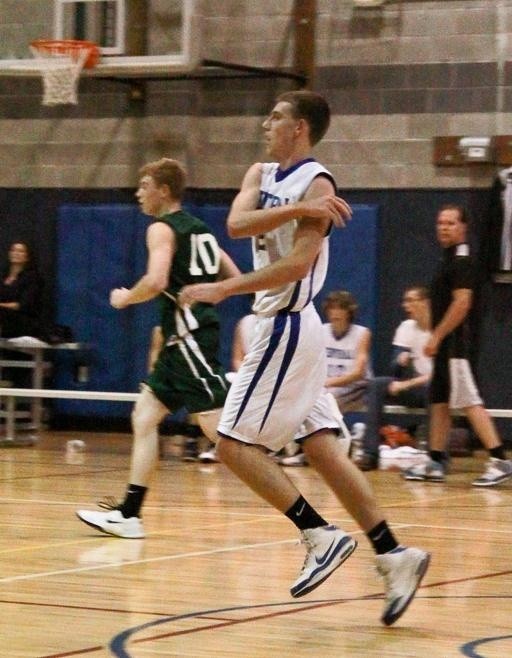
[2,380,511,471]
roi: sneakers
[353,453,378,470]
[471,457,512,486]
[181,440,199,462]
[276,453,308,466]
[373,545,432,625]
[75,497,147,538]
[288,525,358,597]
[400,459,446,481]
[198,447,220,464]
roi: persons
[0,243,42,386]
[76,158,240,539]
[178,90,429,626]
[277,291,371,466]
[198,292,257,464]
[356,282,438,471]
[147,323,198,462]
[401,203,512,488]
